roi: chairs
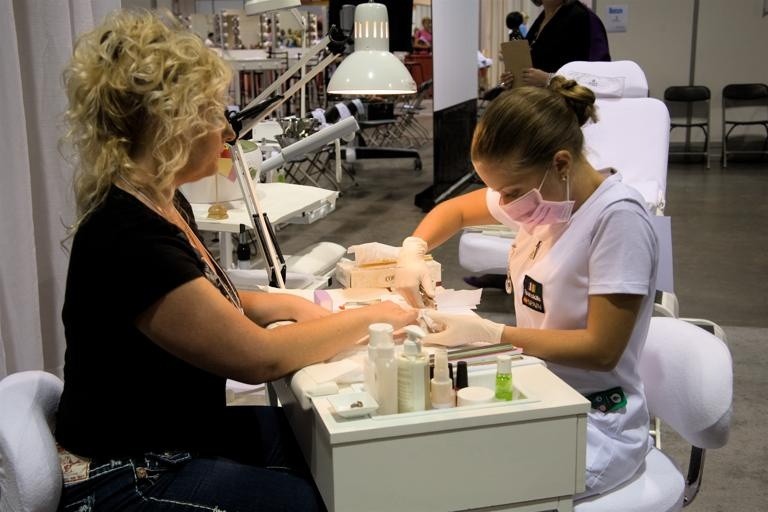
[0,370,66,512]
[277,79,433,196]
[664,84,768,169]
[574,315,733,512]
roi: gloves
[423,309,506,347]
[395,236,435,308]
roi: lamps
[221,3,419,288]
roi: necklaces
[120,175,163,213]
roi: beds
[459,60,670,276]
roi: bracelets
[544,73,554,89]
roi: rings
[500,82,505,88]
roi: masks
[499,162,575,226]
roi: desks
[266,287,592,512]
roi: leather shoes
[463,276,506,288]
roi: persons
[51,8,421,511]
[391,76,660,502]
[482,0,610,89]
[413,16,432,57]
